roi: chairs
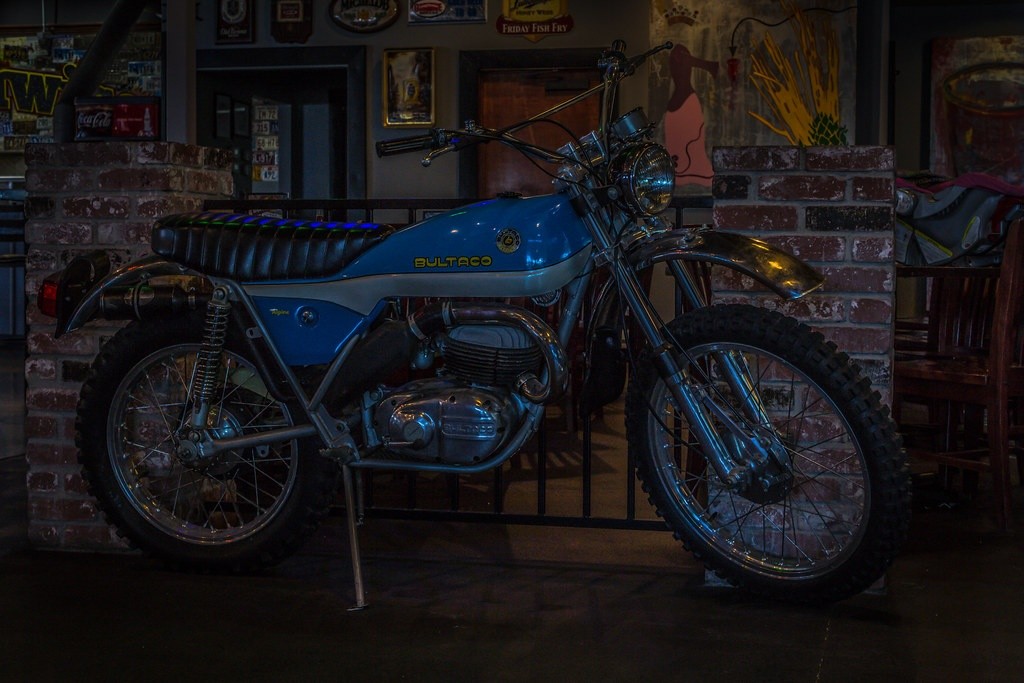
[883,195,1024,530]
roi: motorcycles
[35,38,924,620]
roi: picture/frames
[382,46,437,128]
[213,0,256,45]
[407,1,488,25]
